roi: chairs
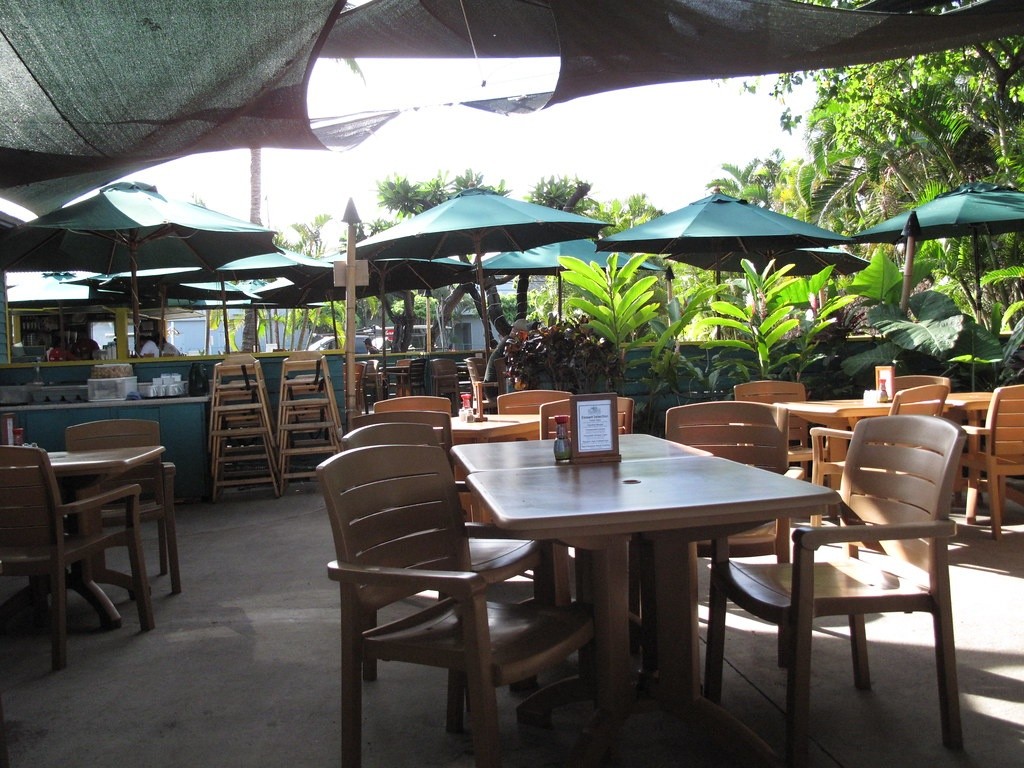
[314,345,1024,768]
[0,416,189,669]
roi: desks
[449,433,715,694]
[452,413,565,522]
[947,391,993,524]
[384,364,408,395]
[765,402,954,535]
[448,457,838,768]
[0,444,164,633]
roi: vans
[307,335,370,353]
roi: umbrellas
[0,181,617,355]
[594,174,1024,339]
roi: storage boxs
[88,376,138,401]
[0,386,32,404]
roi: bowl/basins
[25,356,46,362]
[23,346,46,356]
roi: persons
[47,335,75,361]
[363,338,379,354]
[139,334,180,358]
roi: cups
[137,373,188,397]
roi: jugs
[188,363,210,397]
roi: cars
[371,336,415,353]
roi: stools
[204,348,341,501]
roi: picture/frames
[568,392,620,456]
[875,366,896,400]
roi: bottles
[863,390,878,407]
[13,428,24,446]
[22,443,38,448]
[878,380,888,402]
[459,394,473,423]
[554,417,572,461]
[46,344,64,362]
[21,316,61,350]
[92,338,118,360]
[65,326,88,350]
